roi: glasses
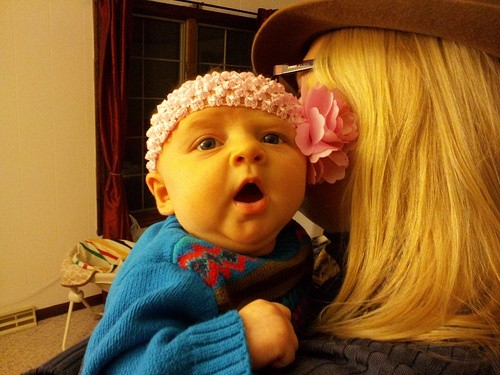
[272,59,315,97]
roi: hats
[250,0,500,94]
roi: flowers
[297,82,365,183]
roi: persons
[79,71,317,375]
[21,0,499,375]
